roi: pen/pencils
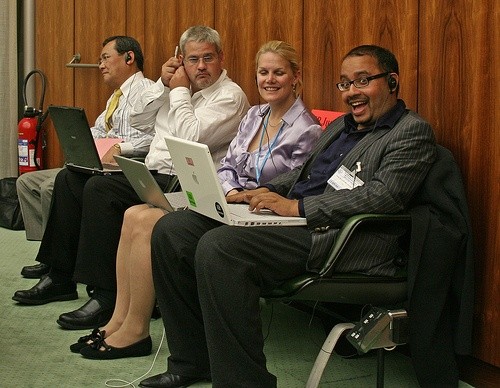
[174,45,179,59]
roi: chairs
[260,146,450,388]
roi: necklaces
[268,117,284,127]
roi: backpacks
[0,177,25,230]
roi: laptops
[164,136,308,226]
[47,105,124,175]
[112,155,190,213]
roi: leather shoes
[138,371,201,388]
[56,297,112,329]
[21,263,47,278]
[12,275,79,304]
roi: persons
[137,45,438,388]
[15,35,158,279]
[12,25,251,330]
[70,40,323,359]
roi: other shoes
[80,329,153,360]
[70,328,107,354]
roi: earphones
[388,77,397,90]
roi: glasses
[184,54,219,67]
[335,70,393,92]
[97,52,125,66]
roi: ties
[104,88,123,132]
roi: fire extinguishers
[16,106,44,175]
